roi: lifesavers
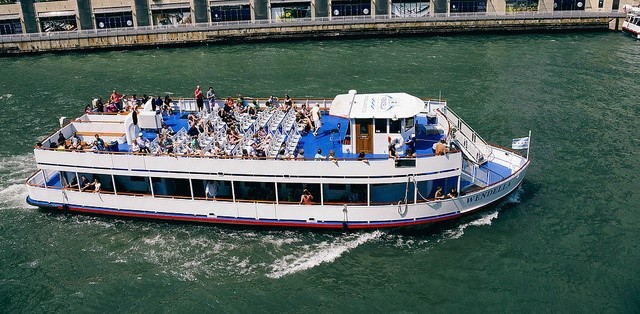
[391,135,403,148]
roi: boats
[622,16,640,39]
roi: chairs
[150,104,309,159]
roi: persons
[435,139,447,155]
[218,94,243,144]
[241,105,257,115]
[163,94,174,116]
[252,101,260,110]
[242,149,248,159]
[70,132,81,144]
[388,143,399,157]
[85,104,93,114]
[194,85,204,110]
[96,97,104,112]
[131,94,140,110]
[141,94,151,107]
[122,94,129,111]
[57,133,66,145]
[157,122,175,157]
[110,90,121,109]
[310,102,322,136]
[406,133,416,155]
[329,150,337,163]
[106,100,119,113]
[357,151,368,163]
[135,132,151,156]
[250,127,273,160]
[186,109,214,155]
[280,143,289,155]
[277,149,290,160]
[296,103,310,134]
[79,175,89,189]
[435,185,445,198]
[215,142,229,159]
[88,177,101,191]
[206,86,215,109]
[204,180,220,198]
[265,101,272,108]
[154,95,163,111]
[91,142,103,151]
[268,95,278,104]
[314,148,327,158]
[297,149,305,161]
[282,94,295,112]
[299,188,313,203]
[95,133,103,146]
[446,185,457,197]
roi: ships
[26,89,532,230]
